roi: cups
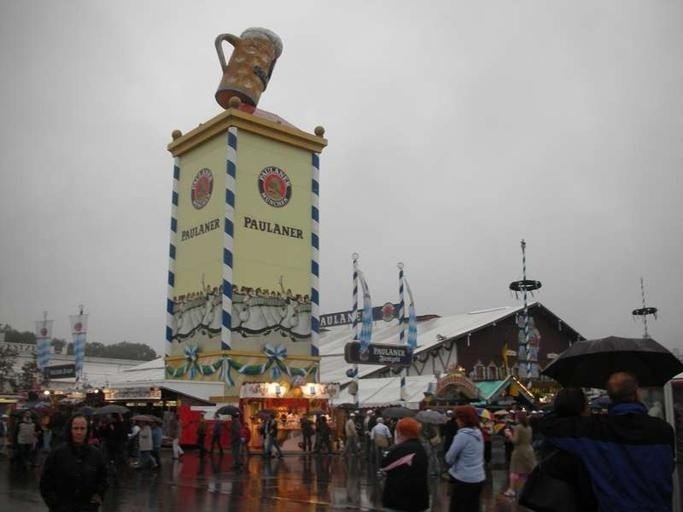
[505,429,510,437]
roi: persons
[300,413,333,455]
[500,411,538,496]
[1,405,164,469]
[378,416,430,511]
[443,405,485,512]
[196,413,207,458]
[38,413,107,512]
[342,407,551,481]
[209,413,225,455]
[229,414,252,467]
[171,415,185,460]
[516,371,678,512]
[261,415,284,461]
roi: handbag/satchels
[483,440,491,463]
[516,448,578,511]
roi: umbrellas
[540,335,683,392]
[302,407,327,416]
[216,402,242,416]
[255,408,279,418]
